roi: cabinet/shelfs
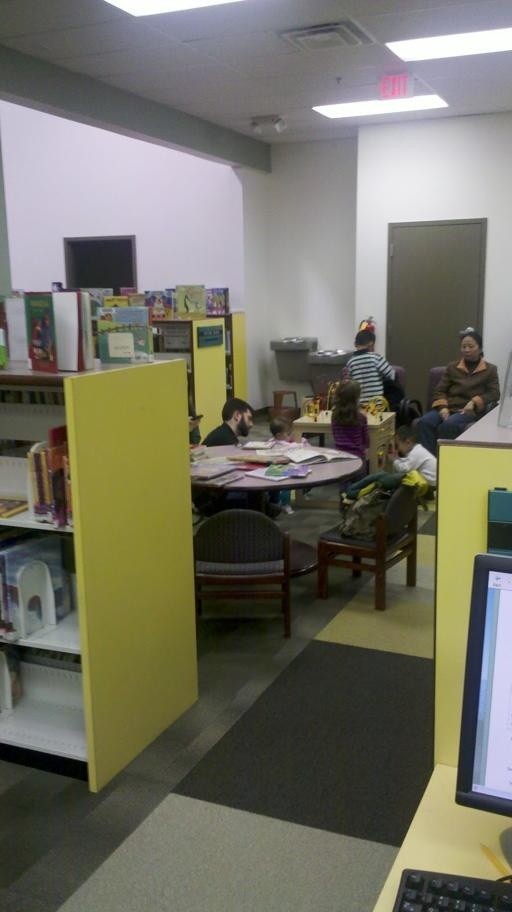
[434,404,511,762]
[0,310,246,792]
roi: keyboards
[392,869,512,912]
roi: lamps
[250,114,287,134]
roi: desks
[370,762,512,912]
[190,445,363,577]
[290,410,396,500]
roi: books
[189,436,361,490]
[0,283,233,447]
[1,643,23,711]
[0,423,72,646]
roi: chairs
[318,483,417,611]
[193,509,291,639]
[343,365,445,426]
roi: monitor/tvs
[454,552,512,818]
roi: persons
[264,416,303,516]
[414,331,501,457]
[331,378,371,512]
[190,398,281,520]
[384,424,437,501]
[343,329,396,413]
[339,339,420,430]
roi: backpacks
[342,489,391,543]
